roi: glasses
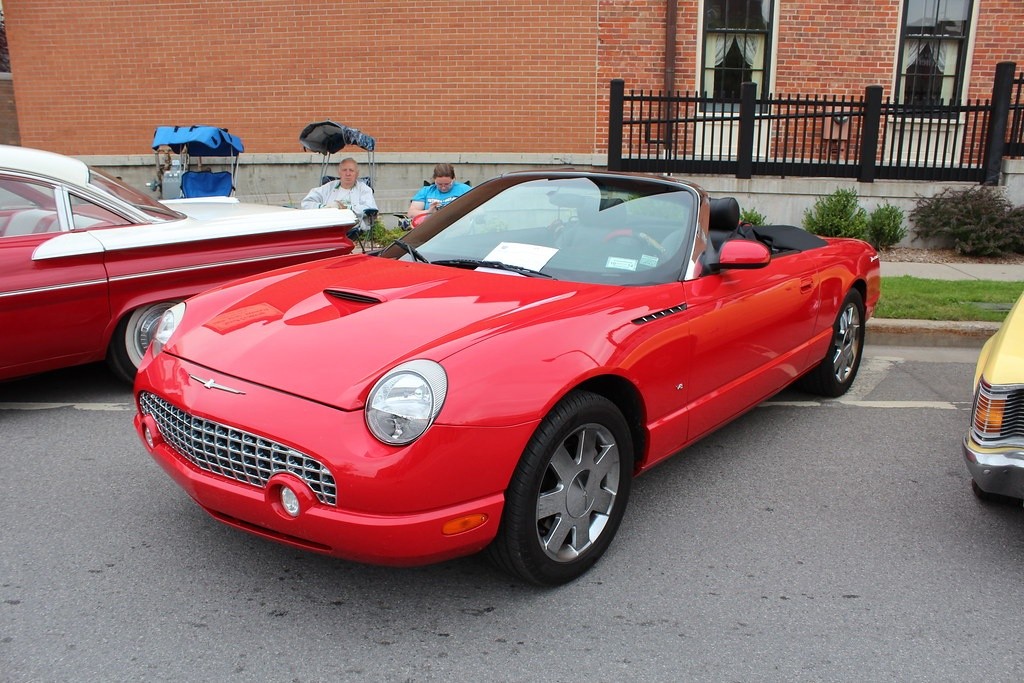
[435,180,454,187]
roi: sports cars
[131,171,883,590]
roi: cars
[0,142,359,391]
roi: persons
[301,157,379,234]
[406,164,473,222]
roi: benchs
[0,209,113,237]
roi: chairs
[552,197,628,267]
[321,176,374,253]
[180,170,233,198]
[657,196,746,275]
